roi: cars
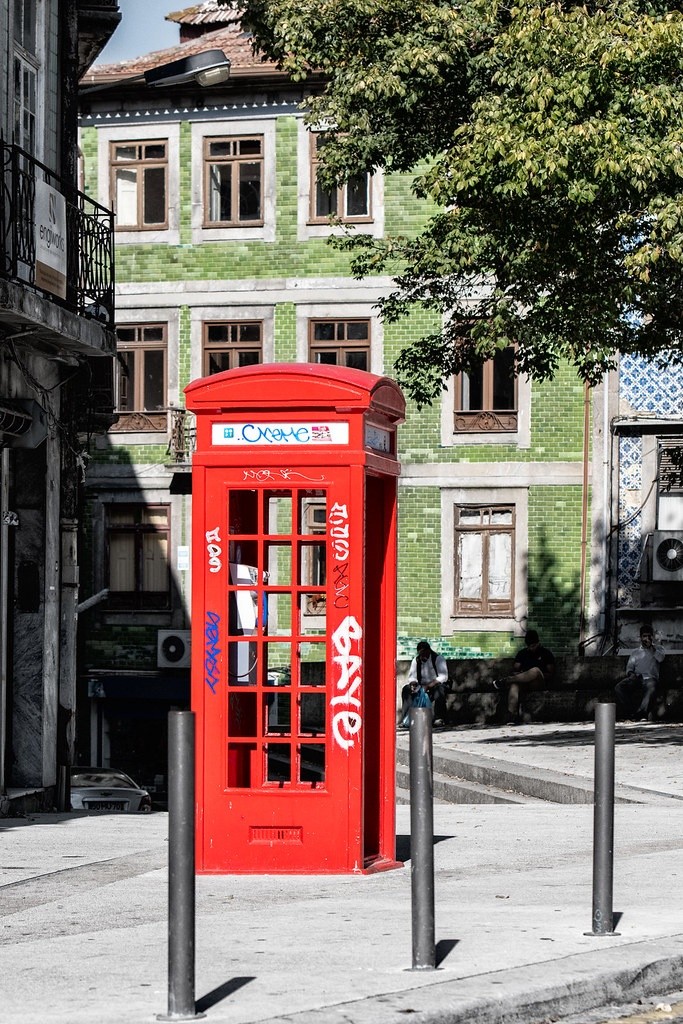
[69,766,152,813]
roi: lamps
[76,49,233,97]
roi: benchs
[439,656,551,719]
[571,653,683,720]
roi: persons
[492,629,555,726]
[614,626,666,722]
[398,641,448,728]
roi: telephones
[229,563,270,636]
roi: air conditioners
[155,627,192,670]
[650,528,683,584]
